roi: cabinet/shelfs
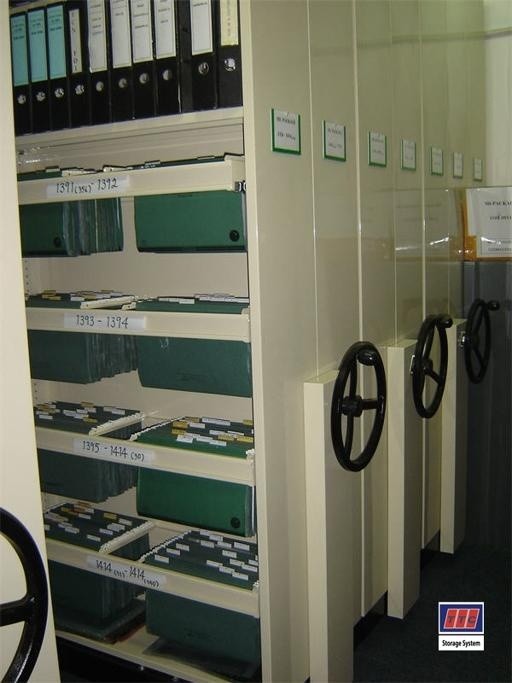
[14,106,259,683]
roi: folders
[9,0,243,136]
